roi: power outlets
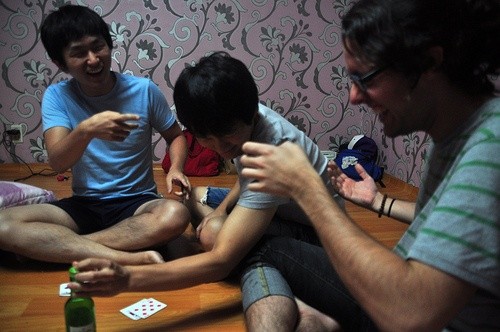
[10,125,24,144]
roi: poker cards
[119,296,167,321]
[58,282,72,297]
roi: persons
[236,0,500,332]
[68,52,344,296]
[0,4,193,270]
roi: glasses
[348,63,398,95]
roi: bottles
[63,267,96,332]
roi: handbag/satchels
[335,136,387,188]
[162,130,220,177]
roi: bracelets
[379,194,387,217]
[388,198,396,217]
